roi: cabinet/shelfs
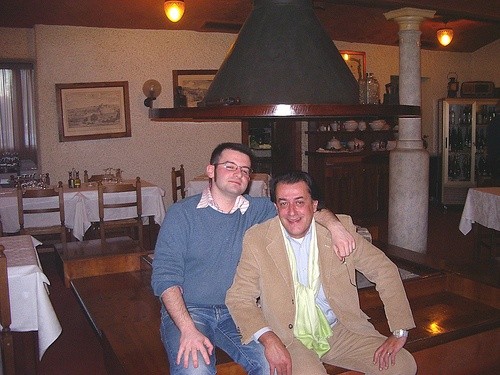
[305,121,400,245]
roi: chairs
[172,164,186,204]
[98,176,143,247]
[10,173,50,187]
[16,180,66,246]
[0,245,16,375]
[84,168,122,183]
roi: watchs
[392,329,408,338]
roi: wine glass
[102,168,116,184]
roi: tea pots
[327,135,342,149]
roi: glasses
[214,162,253,175]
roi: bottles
[84,170,89,183]
[68,170,81,188]
[9,172,51,192]
[334,121,341,130]
[358,73,381,104]
[248,127,272,149]
[450,104,495,176]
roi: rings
[386,352,391,355]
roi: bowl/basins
[344,120,359,131]
[369,120,385,131]
[347,141,365,149]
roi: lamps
[447,72,459,98]
[163,0,185,23]
[142,79,163,108]
[436,18,454,46]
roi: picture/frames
[338,50,367,83]
[55,81,131,143]
[172,70,220,108]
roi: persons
[485,102,500,188]
[150,143,355,375]
[225,171,418,375]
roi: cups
[387,140,397,149]
[371,142,379,150]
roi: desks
[184,173,271,199]
[458,187,500,266]
[0,179,166,375]
